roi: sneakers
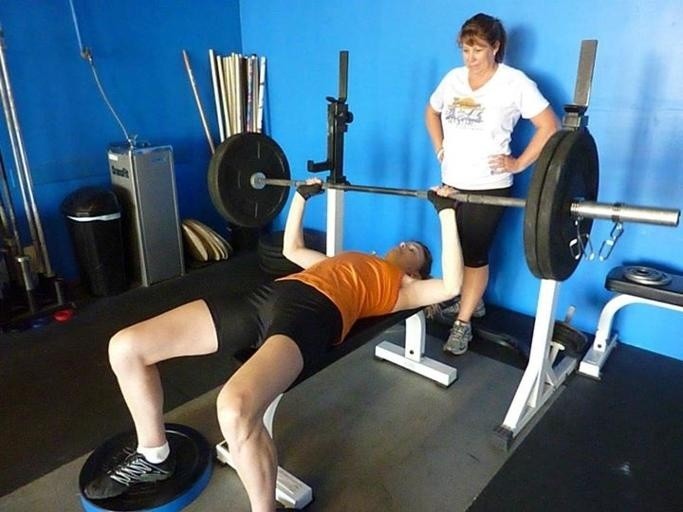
[84,446,177,500]
[443,301,486,317]
[443,319,473,355]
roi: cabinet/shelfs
[108,143,187,290]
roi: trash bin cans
[57,185,134,301]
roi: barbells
[207,130,681,281]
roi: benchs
[576,265,683,381]
[215,276,461,509]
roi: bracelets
[437,148,444,160]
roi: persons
[425,13,562,357]
[82,178,465,512]
